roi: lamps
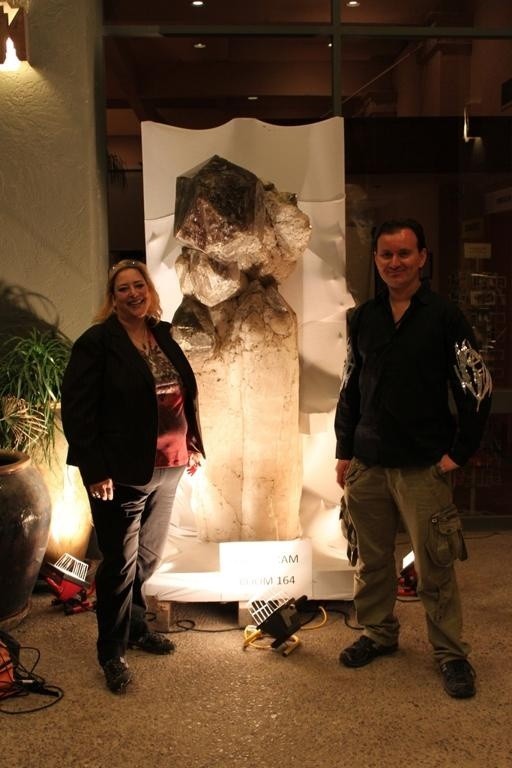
[246,591,317,651]
[45,549,95,616]
[398,551,419,600]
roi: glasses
[112,260,136,275]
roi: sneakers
[339,635,400,669]
[103,658,132,692]
[133,631,177,655]
[440,659,477,700]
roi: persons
[62,256,206,694]
[333,216,492,700]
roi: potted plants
[0,298,71,632]
